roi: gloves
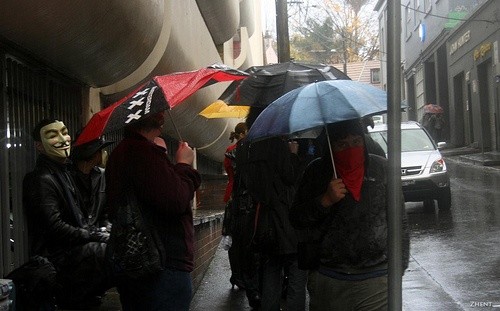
[91,225,111,243]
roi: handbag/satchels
[298,242,320,271]
[222,203,235,237]
[103,147,167,273]
[229,206,276,252]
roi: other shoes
[282,282,288,299]
[251,295,261,311]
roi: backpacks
[8,256,58,311]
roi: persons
[422,113,442,146]
[104,110,200,311]
[223,105,411,311]
[68,128,116,232]
[22,118,115,311]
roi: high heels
[230,276,246,290]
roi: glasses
[159,125,163,131]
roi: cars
[367,121,452,212]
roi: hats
[73,126,116,160]
[320,120,361,142]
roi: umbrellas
[73,60,409,179]
[417,103,444,113]
